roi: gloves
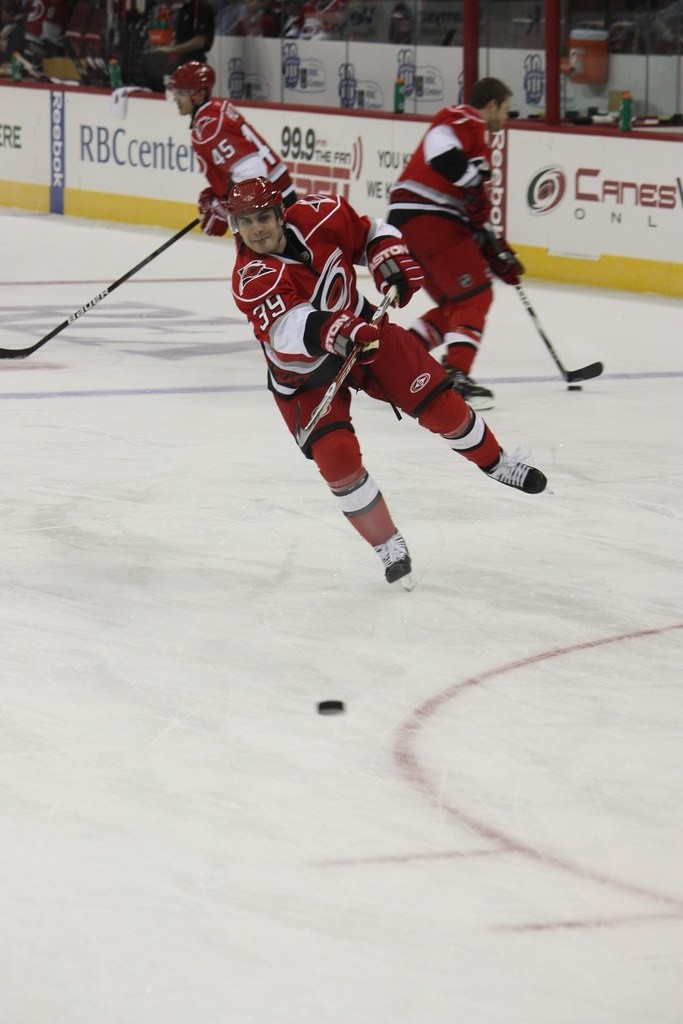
[367,238,425,309]
[198,187,229,236]
[480,238,524,285]
[462,180,492,227]
[320,310,380,365]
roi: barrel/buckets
[566,30,609,86]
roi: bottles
[620,92,634,133]
[109,57,122,90]
[12,50,22,83]
[393,79,405,114]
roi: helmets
[169,60,216,89]
[226,177,283,216]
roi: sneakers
[442,355,494,411]
[478,446,554,494]
[373,527,417,592]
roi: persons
[0,0,415,98]
[227,177,554,591]
[169,61,298,236]
[386,77,525,412]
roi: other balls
[567,385,581,391]
[317,701,344,715]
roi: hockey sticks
[0,214,205,359]
[485,221,604,382]
[293,284,397,450]
